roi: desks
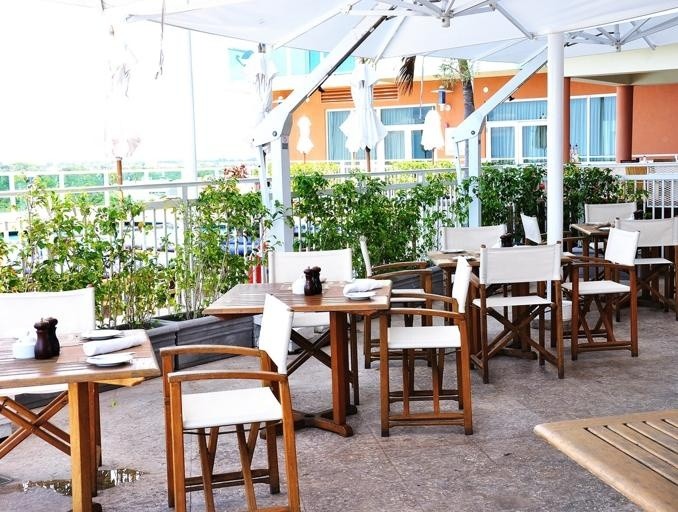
[533,407,678,511]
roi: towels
[341,274,389,293]
[82,332,146,356]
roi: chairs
[548,228,641,362]
[379,255,473,438]
[0,286,102,467]
[584,201,638,280]
[472,240,565,383]
[158,291,301,510]
[359,234,436,371]
[440,223,510,349]
[519,213,589,310]
[611,216,677,325]
[269,247,361,405]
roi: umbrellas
[123,0,678,251]
[420,106,445,164]
[339,56,390,173]
[534,113,548,157]
[419,12,678,228]
[296,114,315,163]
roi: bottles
[313,268,323,295]
[303,265,316,294]
[33,318,53,358]
[47,316,60,357]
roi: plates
[343,282,377,301]
[452,256,474,261]
[320,275,327,284]
[81,330,126,339]
[442,248,464,253]
[597,223,612,230]
[85,353,132,367]
